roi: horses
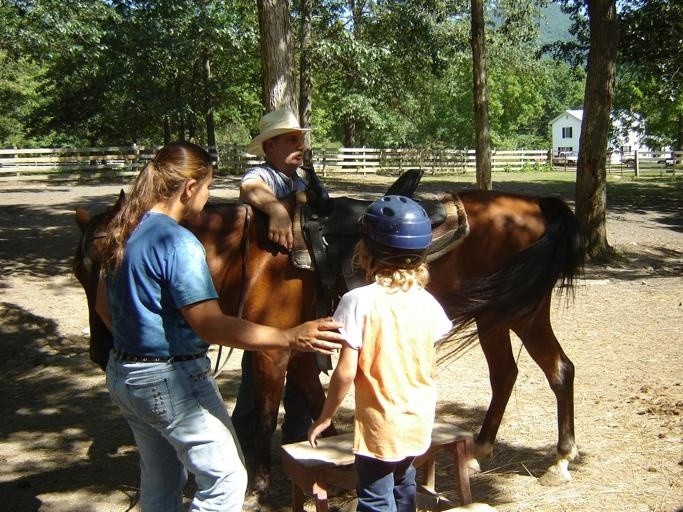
[71,188,587,488]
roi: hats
[246,110,312,156]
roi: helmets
[356,195,433,250]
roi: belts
[117,350,207,362]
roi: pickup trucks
[619,152,677,168]
[553,151,579,166]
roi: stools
[277,420,475,512]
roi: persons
[241,108,314,437]
[305,194,453,511]
[93,144,344,510]
